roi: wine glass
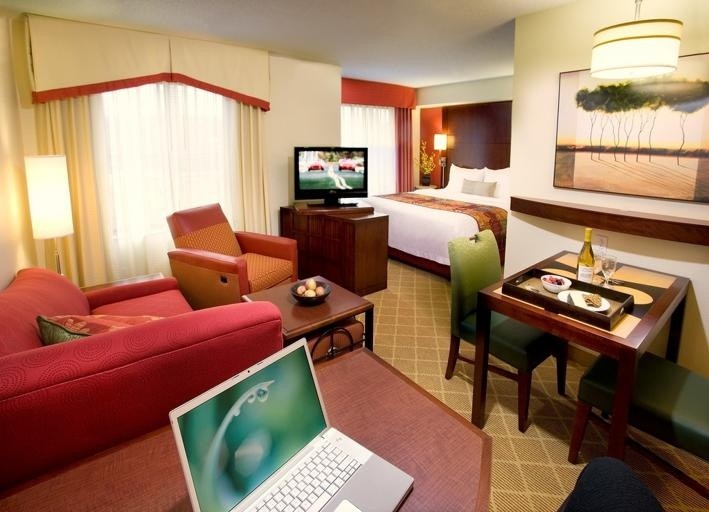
[600,255,617,289]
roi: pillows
[444,163,484,192]
[37,314,164,346]
[460,178,497,198]
[484,167,510,198]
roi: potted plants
[414,139,437,186]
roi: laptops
[169,337,415,512]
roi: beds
[369,186,509,280]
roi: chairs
[445,227,567,432]
[165,202,299,312]
[552,457,666,512]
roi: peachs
[296,277,325,297]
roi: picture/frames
[553,52,709,206]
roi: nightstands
[414,185,439,190]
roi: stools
[567,349,709,464]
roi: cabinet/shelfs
[280,204,390,298]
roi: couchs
[0,266,284,485]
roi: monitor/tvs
[294,147,368,207]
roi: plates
[556,290,611,312]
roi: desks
[3,348,500,510]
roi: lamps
[589,0,685,80]
[434,133,447,188]
[24,152,83,277]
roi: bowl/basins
[289,281,332,304]
[541,274,572,293]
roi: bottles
[576,228,596,284]
